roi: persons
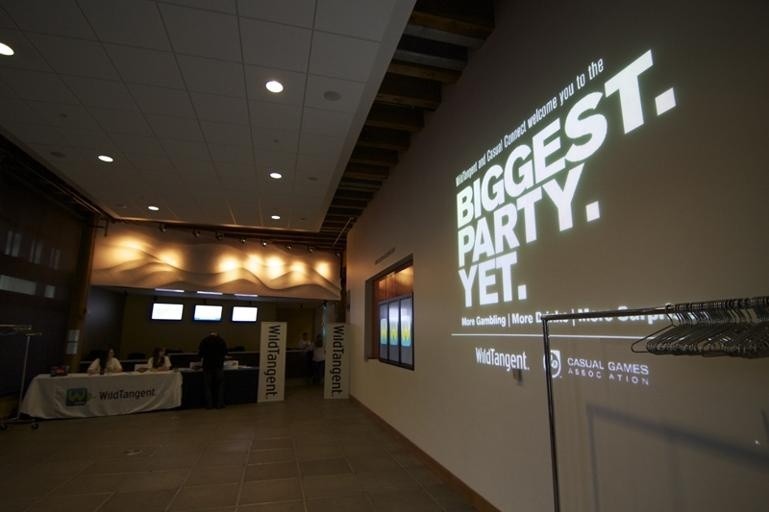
[87,346,123,374]
[298,330,326,386]
[136,347,171,373]
[199,329,229,411]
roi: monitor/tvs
[192,303,224,322]
[150,301,185,321]
[231,305,259,323]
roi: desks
[19,366,258,420]
